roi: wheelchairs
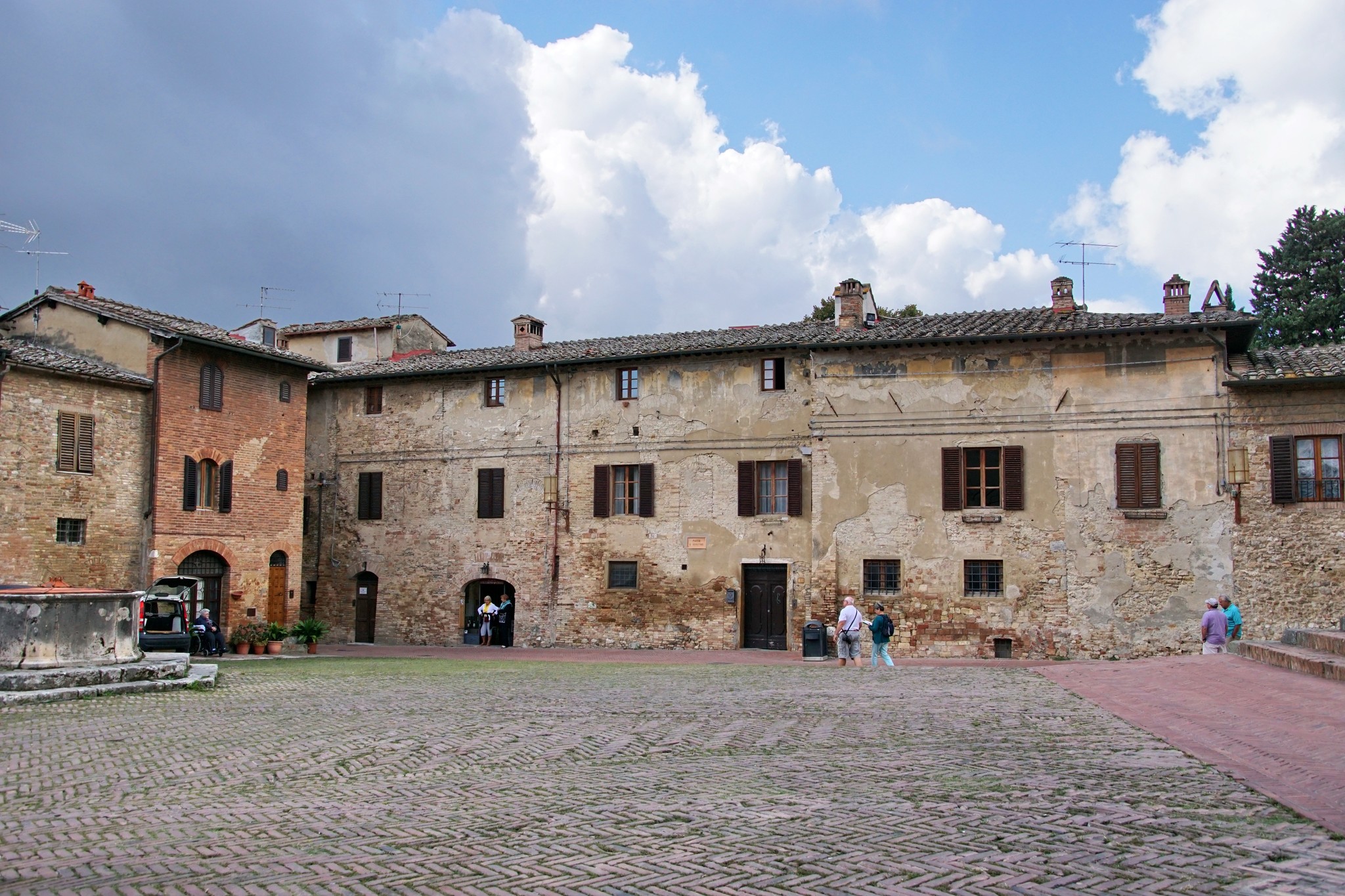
[188,620,225,657]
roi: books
[862,620,872,626]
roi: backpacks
[877,614,894,637]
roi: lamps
[866,313,875,328]
[479,561,490,575]
[543,475,569,535]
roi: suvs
[125,575,203,652]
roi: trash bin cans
[800,621,829,661]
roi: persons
[197,609,230,653]
[1200,598,1228,654]
[1218,593,1242,652]
[833,597,862,667]
[498,594,512,648]
[478,596,499,646]
[867,603,894,666]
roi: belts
[839,629,859,634]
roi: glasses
[204,613,210,615]
[843,600,848,603]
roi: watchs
[1202,638,1206,640]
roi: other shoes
[501,646,506,648]
[221,648,227,650]
[212,648,218,651]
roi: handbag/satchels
[842,635,852,645]
[484,617,489,622]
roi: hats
[1205,598,1218,606]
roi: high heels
[480,642,486,646]
[486,643,490,646]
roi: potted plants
[265,620,291,655]
[231,622,257,654]
[252,623,270,654]
[291,617,330,654]
